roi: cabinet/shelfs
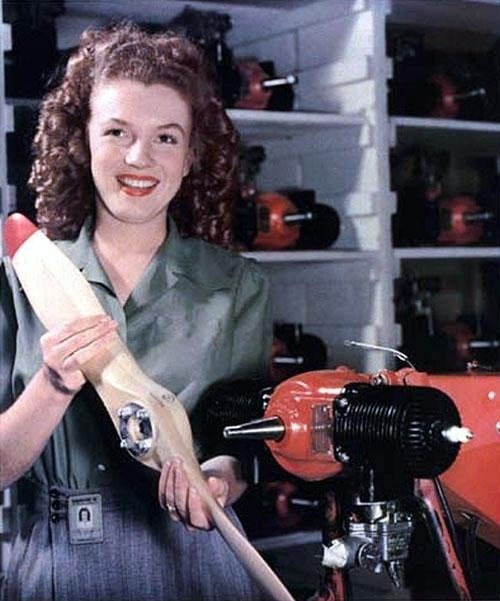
[1,1,500,552]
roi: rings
[167,503,177,513]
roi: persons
[75,507,96,540]
[1,21,272,601]
